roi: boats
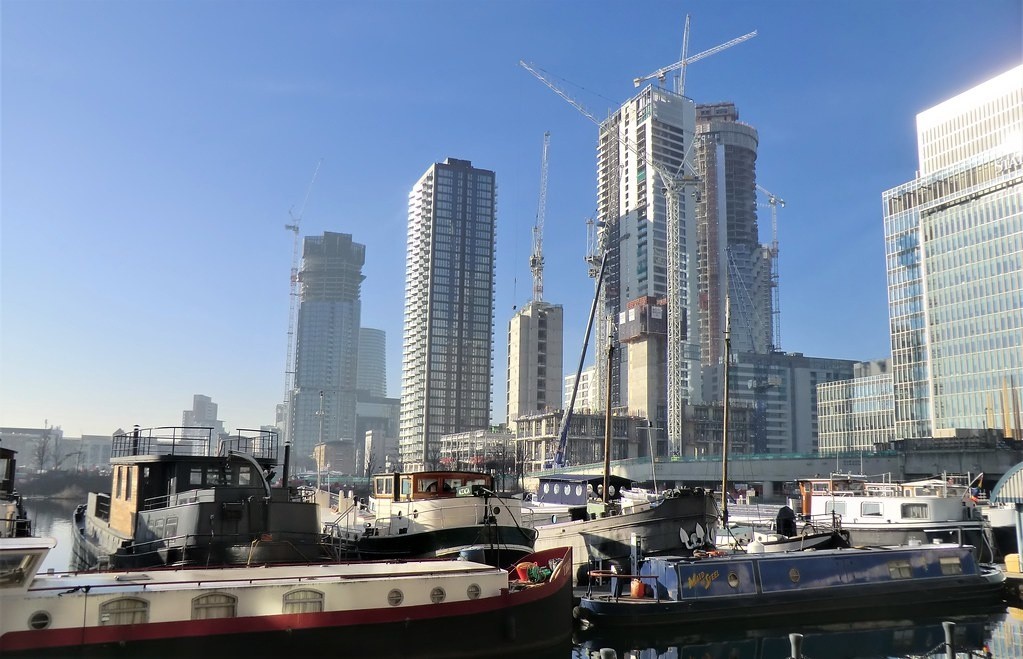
[716,511,851,558]
[520,252,725,597]
[65,423,340,575]
[786,450,996,568]
[297,466,538,583]
[0,446,39,569]
[0,533,578,659]
[565,292,1012,658]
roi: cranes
[285,159,324,479]
[525,128,552,306]
[756,183,784,353]
[721,246,774,460]
[519,55,702,464]
[632,14,761,98]
[587,164,623,413]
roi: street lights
[315,391,325,491]
